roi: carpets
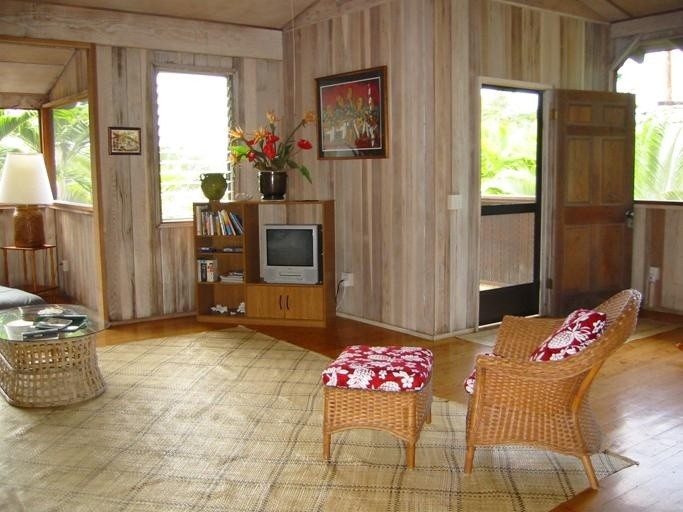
[453,315,682,349]
[1,322,641,511]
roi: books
[17,303,88,342]
[195,205,245,283]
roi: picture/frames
[313,65,388,162]
[108,127,141,155]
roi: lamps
[0,152,55,248]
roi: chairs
[463,288,642,490]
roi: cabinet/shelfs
[194,200,338,328]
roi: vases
[258,170,288,201]
[200,172,228,202]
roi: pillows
[527,307,606,364]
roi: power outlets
[60,260,68,272]
[341,271,354,287]
[648,267,660,282]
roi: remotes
[22,328,59,336]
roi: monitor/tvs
[259,224,322,285]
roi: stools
[0,284,45,312]
[321,343,433,469]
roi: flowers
[226,110,317,185]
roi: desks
[0,302,112,410]
[0,245,60,303]
